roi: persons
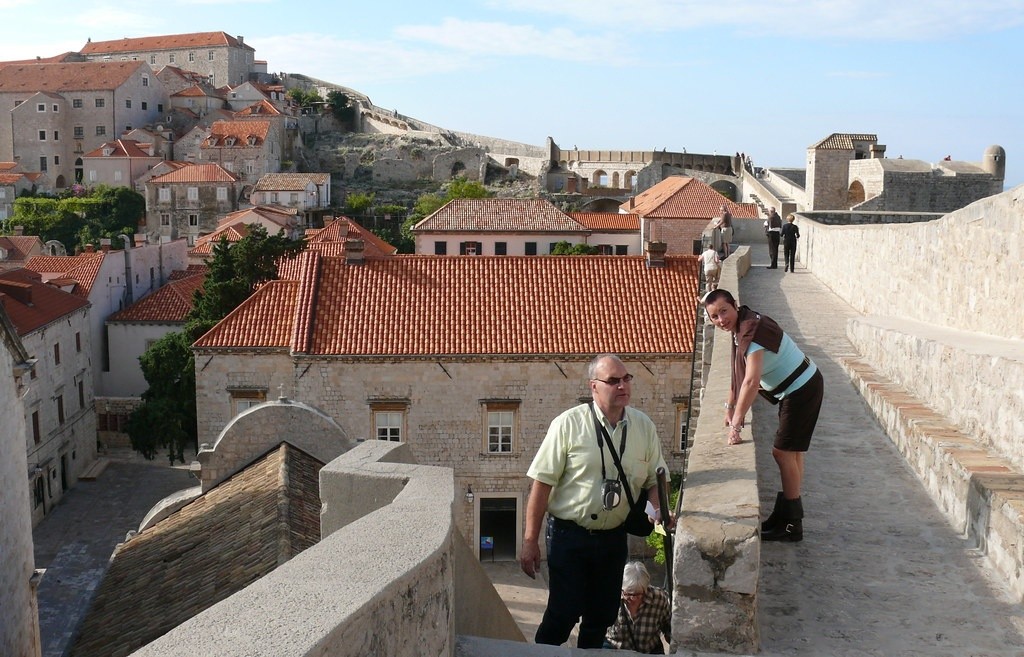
[603,560,672,655]
[478,141,481,149]
[653,147,656,152]
[520,354,675,650]
[662,147,666,152]
[705,289,825,542]
[736,152,740,157]
[741,152,745,161]
[573,145,577,151]
[781,214,800,273]
[763,206,782,270]
[714,205,734,261]
[696,283,718,323]
[697,245,719,292]
[446,129,451,140]
[682,147,686,153]
[392,108,397,118]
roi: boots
[760,492,806,532]
[762,500,803,542]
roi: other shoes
[785,265,788,272]
[790,268,794,272]
[767,266,777,269]
[719,256,727,260]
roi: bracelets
[724,403,734,409]
[730,425,741,431]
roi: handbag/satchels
[625,488,653,537]
[757,383,779,405]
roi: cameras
[600,478,621,512]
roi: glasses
[595,373,634,386]
[622,590,645,597]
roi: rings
[732,438,736,442]
